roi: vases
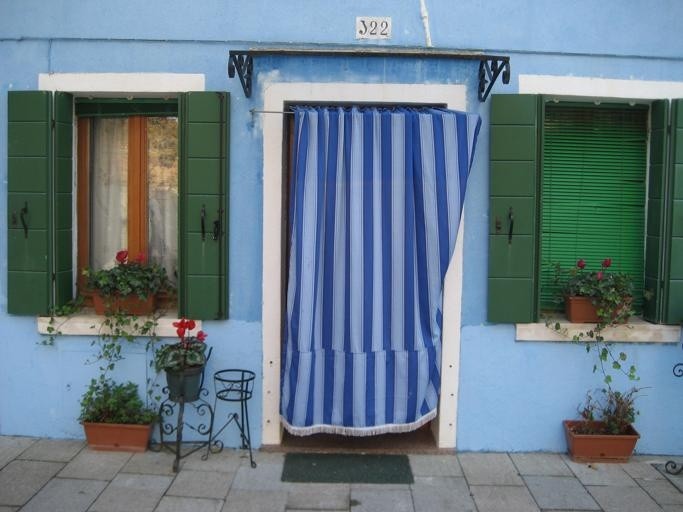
[93,285,162,317]
[564,294,636,325]
[562,417,638,468]
[159,361,206,402]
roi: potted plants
[77,379,166,454]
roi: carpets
[280,451,413,486]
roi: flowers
[147,318,211,373]
[571,383,643,435]
[34,246,180,415]
[535,252,658,387]
